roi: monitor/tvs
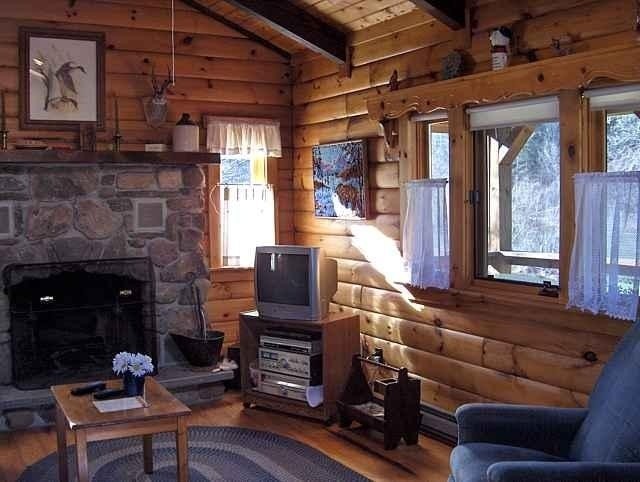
[253,244,338,321]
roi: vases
[123,372,145,395]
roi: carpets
[14,425,370,482]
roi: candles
[1,91,6,130]
[115,97,119,135]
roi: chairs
[447,319,640,482]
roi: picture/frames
[310,137,369,221]
[18,25,107,132]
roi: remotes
[71,381,107,396]
[93,387,126,399]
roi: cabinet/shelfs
[239,309,361,420]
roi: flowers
[112,351,155,374]
